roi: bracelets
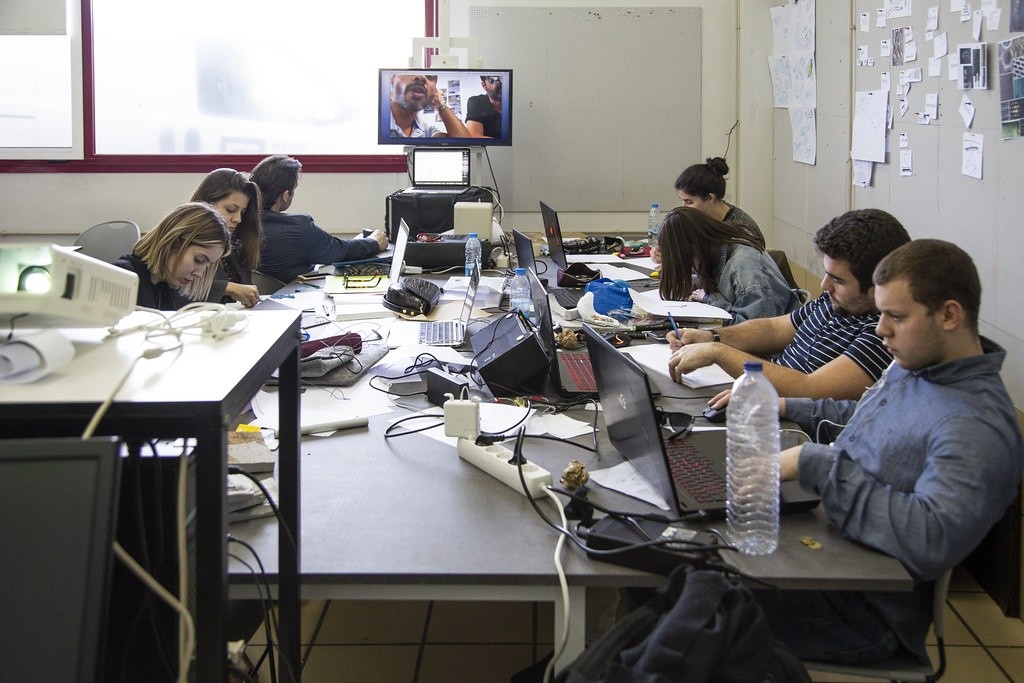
[438,105,447,112]
[704,329,720,342]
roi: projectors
[0,243,139,330]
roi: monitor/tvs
[377,69,513,146]
[0,436,122,683]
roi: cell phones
[298,272,332,280]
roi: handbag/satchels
[301,345,356,378]
[384,276,444,316]
[586,277,632,321]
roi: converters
[585,513,719,577]
[427,367,469,409]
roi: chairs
[806,496,1014,683]
[79,219,141,262]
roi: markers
[623,332,647,339]
[632,322,680,332]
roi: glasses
[342,272,381,287]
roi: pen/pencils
[668,312,681,340]
[323,305,329,316]
[303,283,320,288]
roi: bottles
[648,204,662,242]
[464,233,482,278]
[724,359,781,556]
[509,268,531,322]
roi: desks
[196,233,913,682]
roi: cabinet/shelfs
[0,308,302,683]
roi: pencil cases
[602,236,623,253]
[562,236,601,255]
[556,262,603,287]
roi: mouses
[702,405,728,424]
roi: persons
[110,203,230,312]
[248,154,389,284]
[648,156,765,264]
[509,239,1023,683]
[465,76,503,140]
[191,166,262,308]
[665,209,913,446]
[389,75,471,139]
[656,207,802,328]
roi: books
[627,288,732,331]
[616,343,738,387]
[441,275,507,308]
[226,427,280,520]
[329,293,397,320]
[269,399,368,439]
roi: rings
[252,299,256,301]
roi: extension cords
[457,437,554,499]
[496,252,512,267]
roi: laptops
[387,259,480,348]
[401,147,472,194]
[328,218,410,298]
[512,201,823,518]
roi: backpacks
[557,564,812,683]
